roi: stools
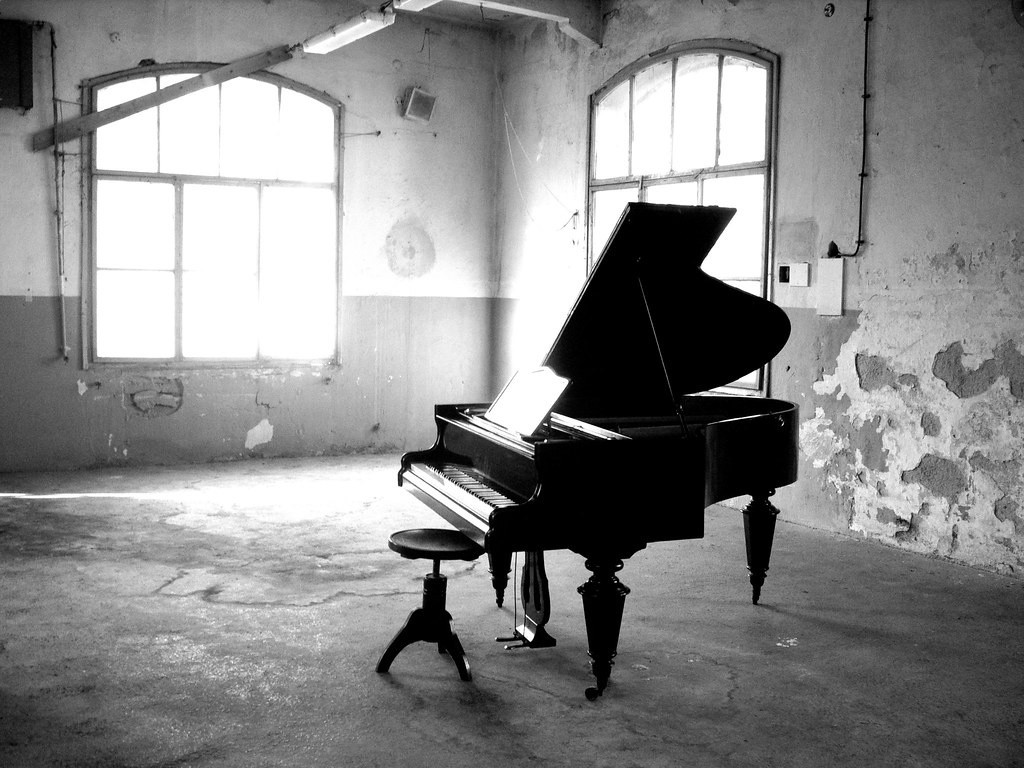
[373,529,487,682]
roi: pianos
[400,201,800,701]
